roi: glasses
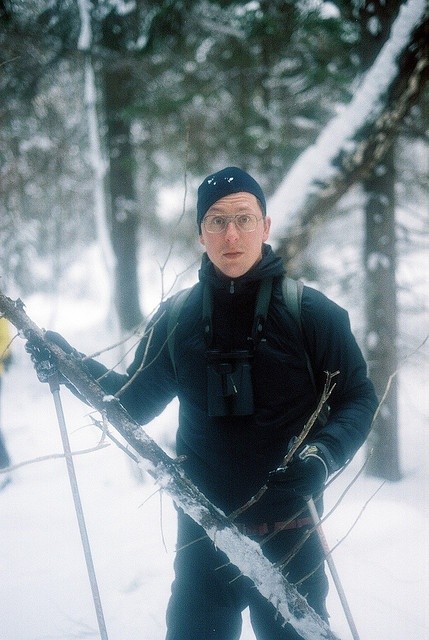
[203,213,264,232]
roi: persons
[23,165,380,636]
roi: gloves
[25,328,83,387]
[266,437,344,498]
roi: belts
[229,516,315,537]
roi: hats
[197,167,267,232]
